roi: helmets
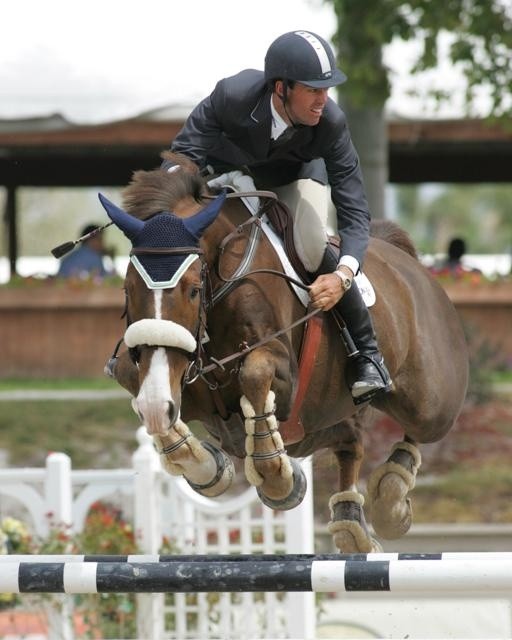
[265,29,348,88]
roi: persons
[431,237,466,271]
[55,225,107,278]
[97,30,398,401]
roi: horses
[96,150,470,553]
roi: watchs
[333,270,352,292]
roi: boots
[305,243,394,398]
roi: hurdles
[1,548,512,593]
